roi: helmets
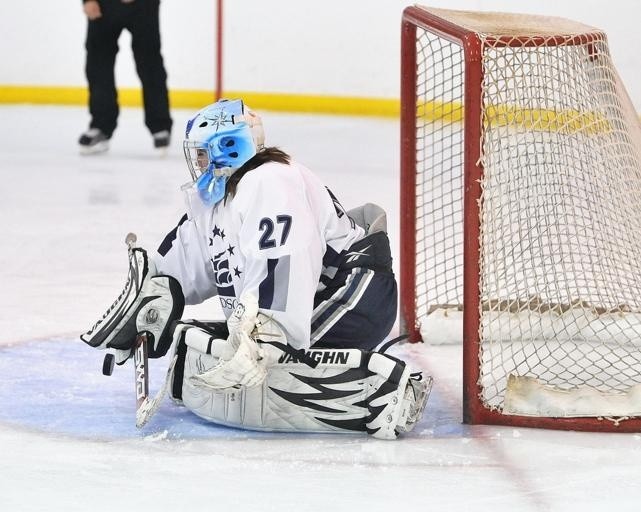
[183,100,265,173]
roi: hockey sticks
[123,233,177,429]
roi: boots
[154,131,172,146]
[78,128,110,145]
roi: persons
[71,0,174,149]
[76,94,425,442]
